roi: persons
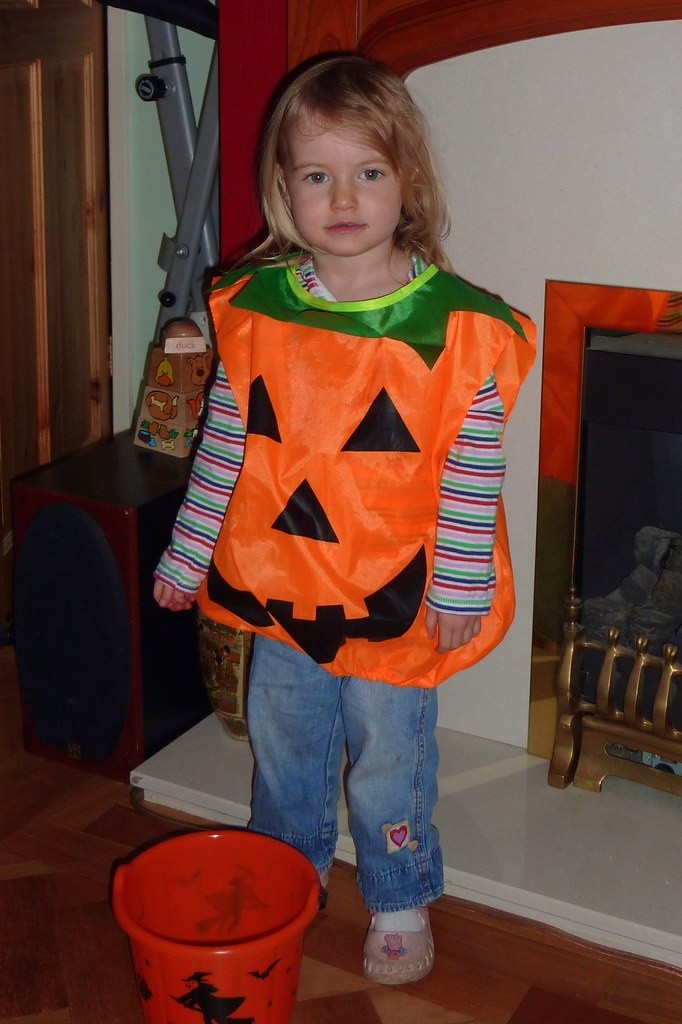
[154,56,539,984]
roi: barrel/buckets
[110,825,321,1024]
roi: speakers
[12,429,198,784]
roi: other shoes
[362,906,434,984]
